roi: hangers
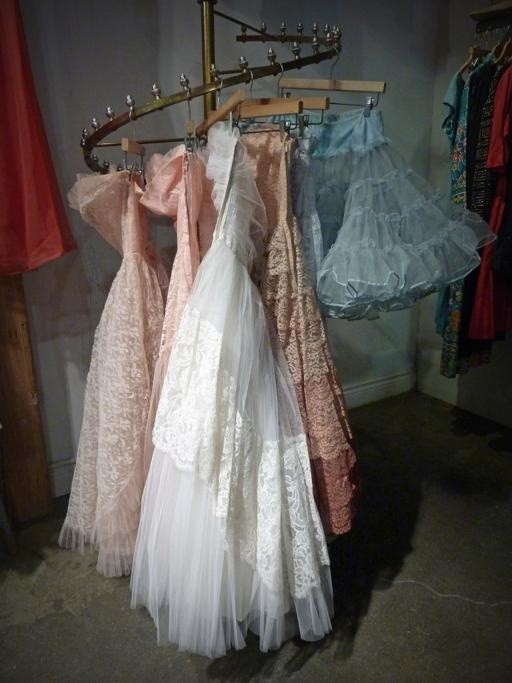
[112,43,389,171]
[460,22,512,75]
